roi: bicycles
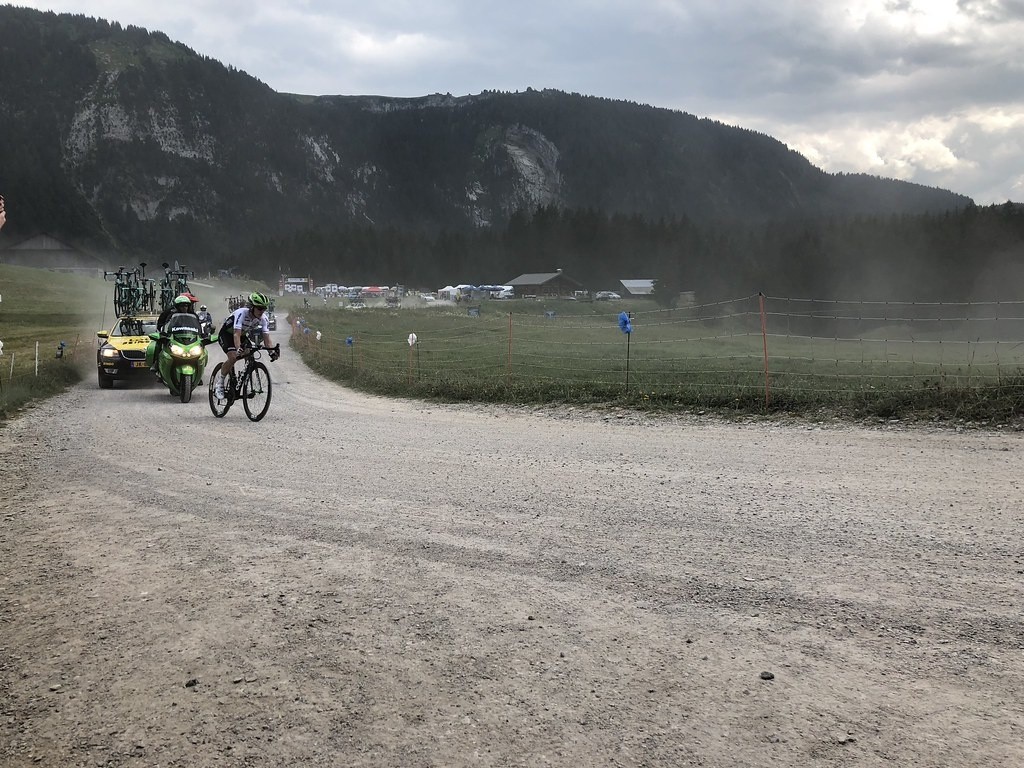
[225,293,276,312]
[208,341,280,422]
[104,259,194,319]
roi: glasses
[201,309,206,310]
[178,303,189,307]
[255,307,268,310]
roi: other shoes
[150,363,158,372]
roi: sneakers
[215,382,225,399]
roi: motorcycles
[196,311,216,345]
[146,313,218,403]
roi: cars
[344,303,366,310]
[383,303,401,309]
[421,293,435,302]
[385,294,401,303]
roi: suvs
[349,294,367,303]
[596,291,621,301]
[264,313,278,331]
[96,313,161,388]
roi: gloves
[236,347,244,356]
[269,350,280,360]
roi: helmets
[172,295,192,303]
[247,292,270,307]
[200,305,207,309]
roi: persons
[214,291,279,399]
[150,292,212,385]
[304,297,326,308]
